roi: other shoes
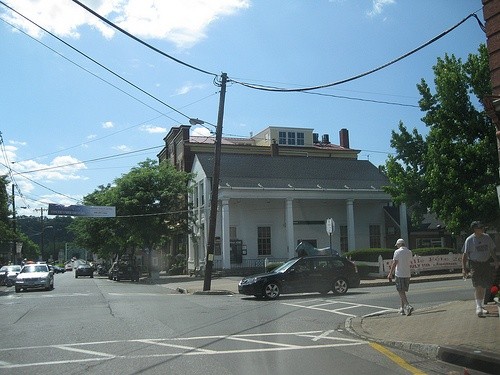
[476,308,482,317]
[406,305,413,316]
[398,308,405,315]
[483,307,489,314]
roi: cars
[53,266,64,274]
[0,265,21,281]
[26,257,76,270]
[108,262,125,280]
[89,261,107,277]
[15,263,54,292]
[65,264,73,271]
[75,264,93,279]
[113,264,139,282]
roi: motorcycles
[0,269,13,287]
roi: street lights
[41,226,53,260]
[189,118,224,292]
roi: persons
[462,221,498,316]
[388,239,414,316]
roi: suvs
[238,254,360,300]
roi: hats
[471,221,484,230]
[395,239,405,246]
